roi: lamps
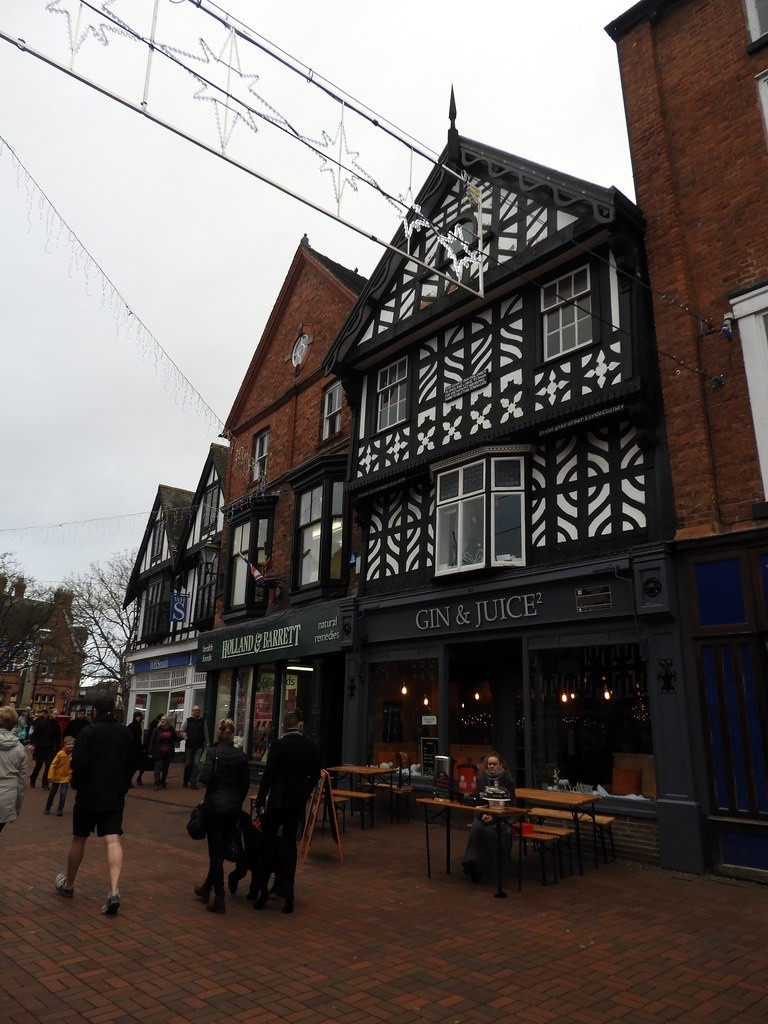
[199,540,224,589]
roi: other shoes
[281,894,295,914]
[206,903,226,914]
[228,871,239,894]
[247,883,258,901]
[194,884,211,903]
[253,887,270,911]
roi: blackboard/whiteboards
[305,769,326,840]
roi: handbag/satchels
[223,816,258,865]
[186,799,208,840]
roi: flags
[240,552,265,584]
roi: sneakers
[101,891,122,914]
[56,874,75,897]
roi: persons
[43,735,76,816]
[179,705,211,790]
[54,695,133,915]
[5,699,93,791]
[0,704,28,843]
[460,752,516,886]
[137,713,171,786]
[255,708,320,919]
[227,802,272,909]
[126,711,145,787]
[194,719,250,914]
[149,714,182,790]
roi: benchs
[321,795,349,834]
[355,781,414,824]
[533,824,575,880]
[249,794,268,819]
[322,788,376,830]
[467,824,560,886]
[528,808,617,866]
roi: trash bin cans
[420,737,440,777]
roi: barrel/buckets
[433,755,456,799]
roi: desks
[326,764,399,825]
[417,798,532,899]
[515,787,603,878]
